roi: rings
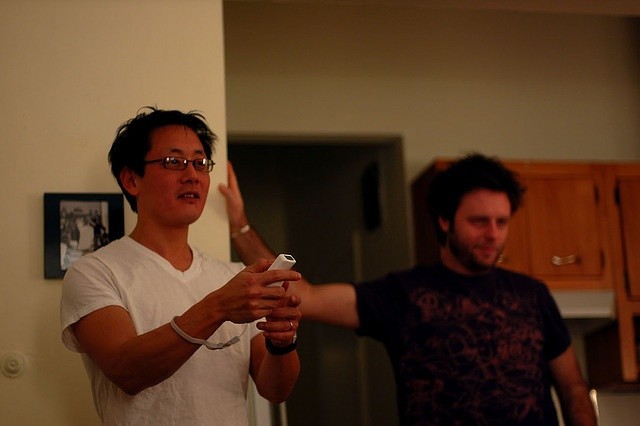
[292,332,297,344]
[289,321,293,332]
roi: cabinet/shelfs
[587,157,635,392]
[433,158,609,287]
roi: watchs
[265,336,296,356]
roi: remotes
[263,254,297,293]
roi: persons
[218,155,599,426]
[59,109,301,426]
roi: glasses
[138,156,217,171]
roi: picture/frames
[42,191,124,280]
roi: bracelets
[223,222,252,241]
[170,316,208,351]
